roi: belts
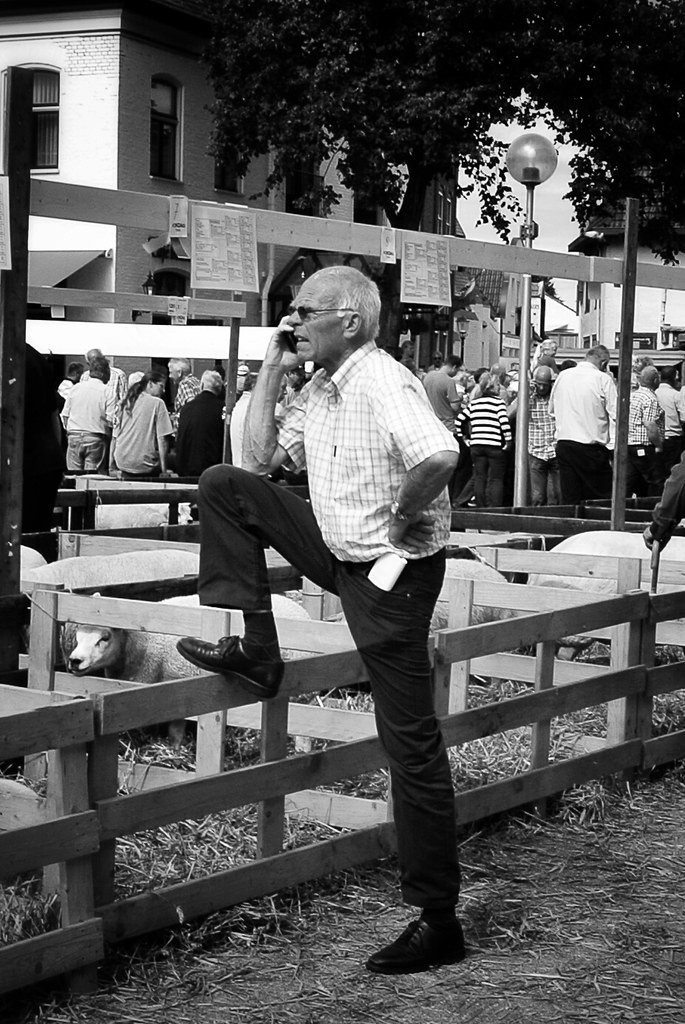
[66,428,106,438]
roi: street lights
[504,129,560,520]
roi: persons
[177,265,467,973]
[59,349,309,483]
[375,337,685,510]
[642,450,685,787]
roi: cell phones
[286,330,298,354]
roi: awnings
[29,250,107,287]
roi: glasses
[286,305,355,320]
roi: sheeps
[524,531,685,649]
[67,591,319,756]
[19,544,201,671]
[287,555,516,690]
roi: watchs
[391,500,417,521]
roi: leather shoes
[363,920,470,974]
[176,635,285,698]
